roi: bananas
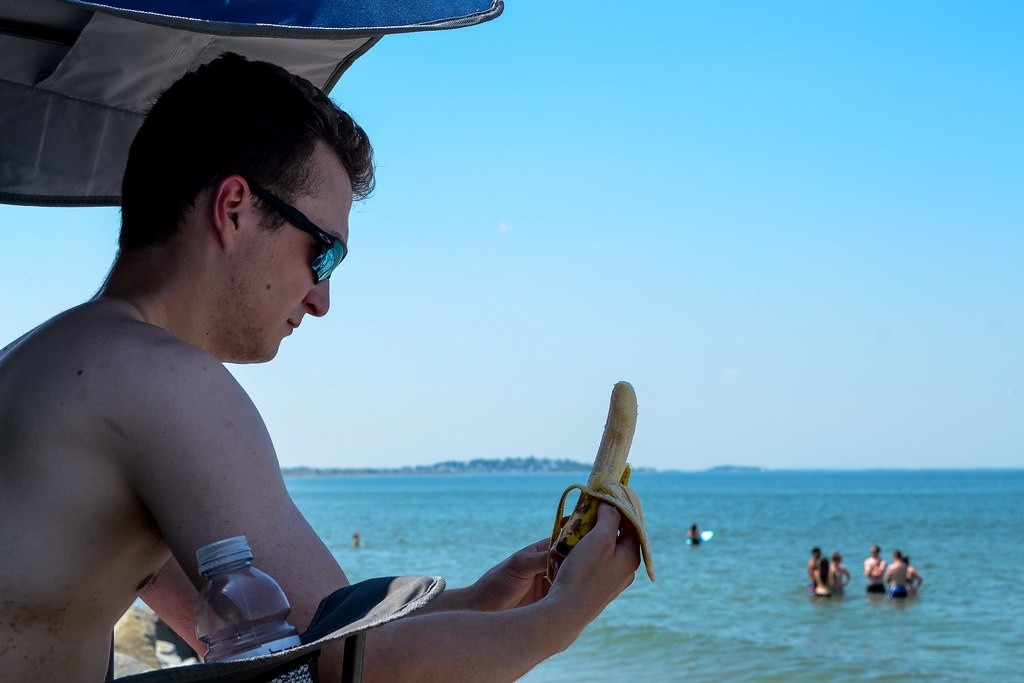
[545,380,654,585]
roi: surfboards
[686,531,713,544]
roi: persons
[353,534,360,546]
[0,52,641,683]
[685,524,925,606]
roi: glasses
[248,182,348,285]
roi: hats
[107,575,446,683]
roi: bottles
[193,533,309,683]
